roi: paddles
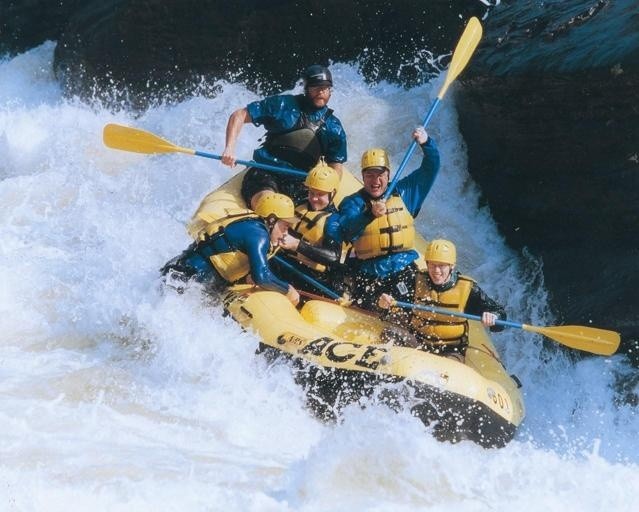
[103,124,309,180]
[390,301,620,356]
[382,17,483,206]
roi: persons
[245,164,343,301]
[219,64,347,212]
[325,127,442,309]
[156,192,301,313]
[372,237,509,367]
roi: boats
[183,158,528,449]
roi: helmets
[424,239,457,264]
[305,65,332,89]
[255,193,295,218]
[303,165,340,193]
[360,149,390,171]
